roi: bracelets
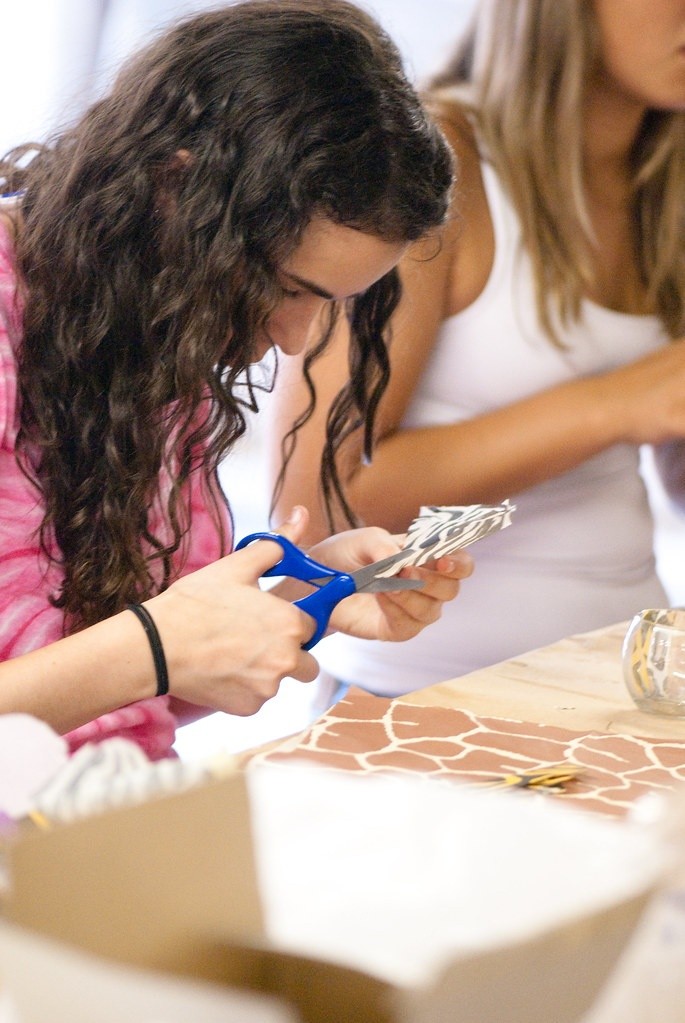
[123,599,171,697]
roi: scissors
[235,532,425,651]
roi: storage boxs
[1,759,658,1023]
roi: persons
[0,1,476,764]
[272,0,685,721]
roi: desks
[252,618,685,857]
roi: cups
[620,608,684,717]
